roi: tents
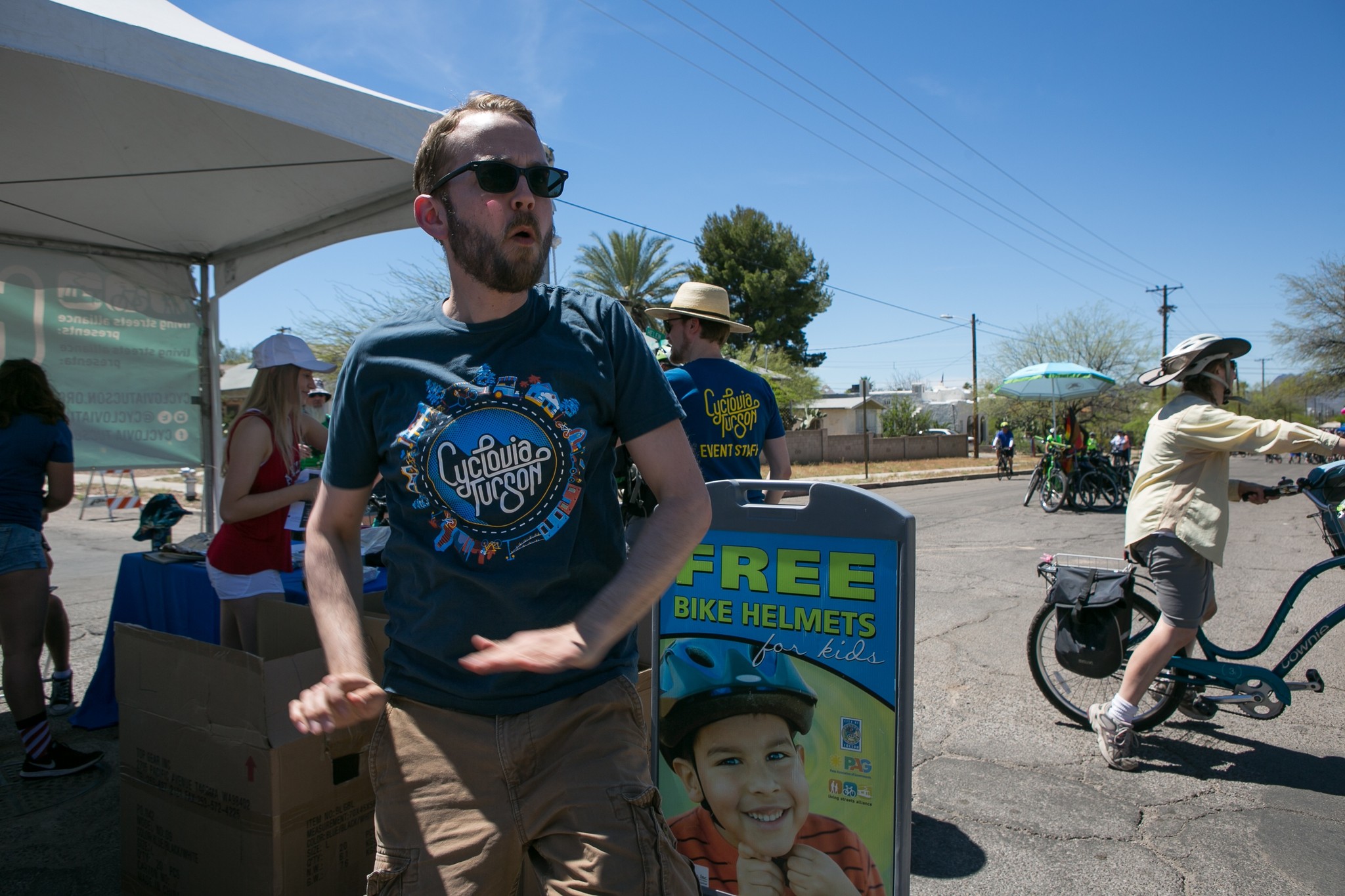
[1,0,564,538]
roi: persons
[286,91,714,896]
[616,282,794,666]
[991,421,1014,473]
[1088,334,1345,772]
[203,333,382,655]
[656,635,887,896]
[1044,426,1130,469]
[0,361,109,777]
[1235,450,1318,464]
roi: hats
[309,377,331,402]
[252,334,337,373]
[132,493,194,541]
[644,282,753,334]
[1137,338,1252,386]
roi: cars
[918,428,975,444]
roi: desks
[67,535,393,734]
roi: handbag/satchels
[1307,458,1345,541]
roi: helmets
[1160,335,1226,375]
[658,637,820,770]
[656,345,685,367]
[1000,421,1008,427]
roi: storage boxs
[114,594,391,896]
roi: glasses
[663,318,683,334]
[1231,360,1237,369]
[433,160,569,199]
[309,394,324,398]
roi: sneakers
[1088,702,1141,771]
[1149,670,1214,721]
[21,742,105,779]
[50,670,73,716]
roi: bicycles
[1024,458,1345,734]
[1231,450,1345,465]
[993,445,1014,481]
[1023,435,1137,513]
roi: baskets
[1307,505,1345,569]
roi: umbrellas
[992,362,1117,442]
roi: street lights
[940,312,979,459]
